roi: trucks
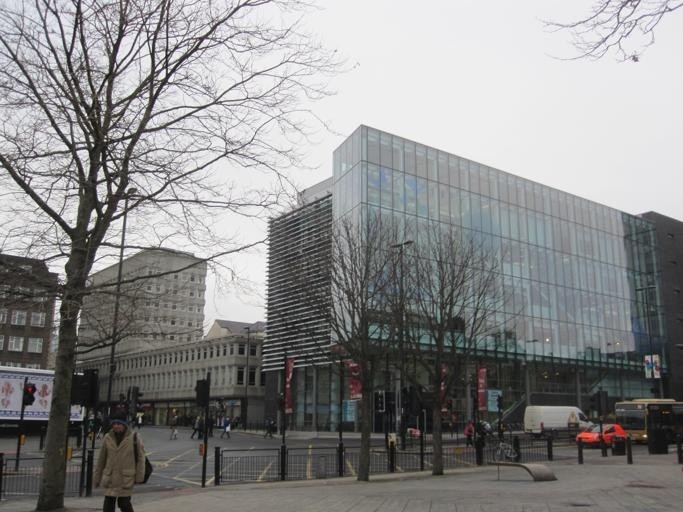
[523,406,596,442]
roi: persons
[461,420,476,448]
[93,413,145,512]
[118,409,287,443]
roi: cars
[575,423,630,449]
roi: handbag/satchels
[134,454,154,485]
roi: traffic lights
[196,378,210,408]
[71,371,101,409]
[471,397,481,411]
[447,399,452,410]
[130,386,146,414]
[496,396,505,410]
[373,390,386,412]
[23,384,39,407]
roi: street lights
[106,186,139,425]
[635,286,658,399]
[605,341,621,375]
[525,338,539,380]
[390,237,415,452]
[243,327,252,432]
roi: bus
[613,396,683,447]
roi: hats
[109,410,129,429]
